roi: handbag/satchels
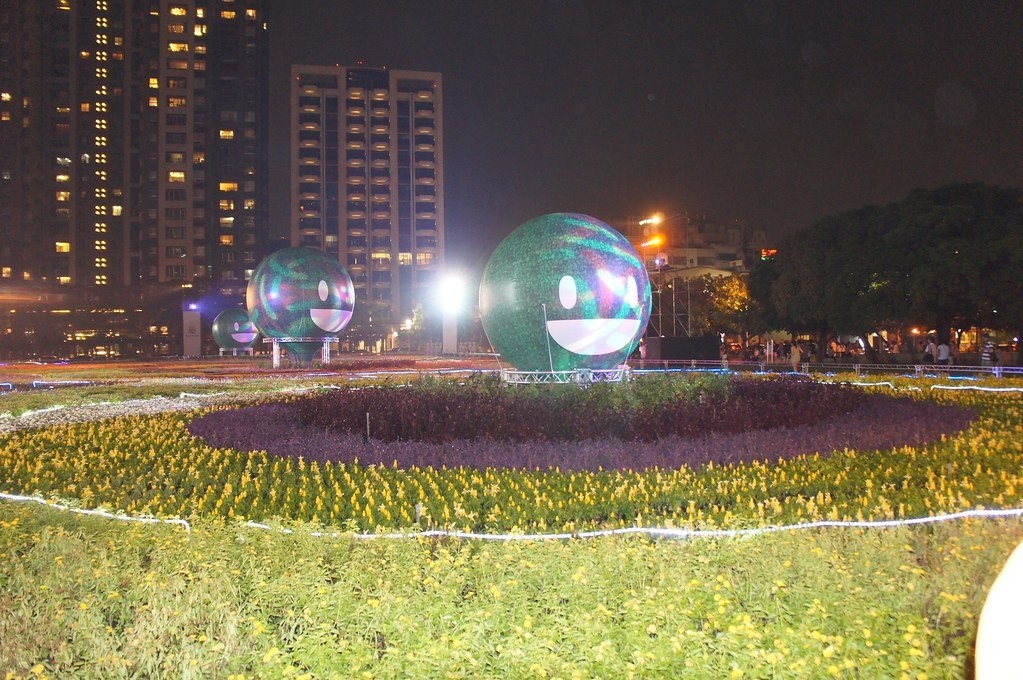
[923,353,934,362]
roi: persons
[639,343,646,358]
[719,330,1023,374]
